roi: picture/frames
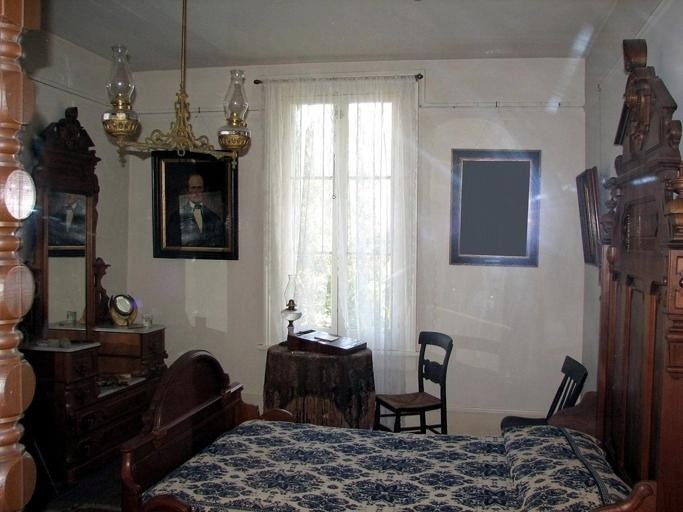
[48,188,86,259]
[152,150,239,260]
[449,150,542,268]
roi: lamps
[279,300,302,345]
[102,1,251,169]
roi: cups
[65,311,77,323]
[142,313,153,328]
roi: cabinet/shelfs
[17,325,169,495]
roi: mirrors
[41,188,93,342]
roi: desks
[263,345,373,426]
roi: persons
[179,173,226,249]
[49,192,85,246]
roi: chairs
[501,356,588,434]
[375,330,452,433]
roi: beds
[121,350,683,510]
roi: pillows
[501,425,631,510]
